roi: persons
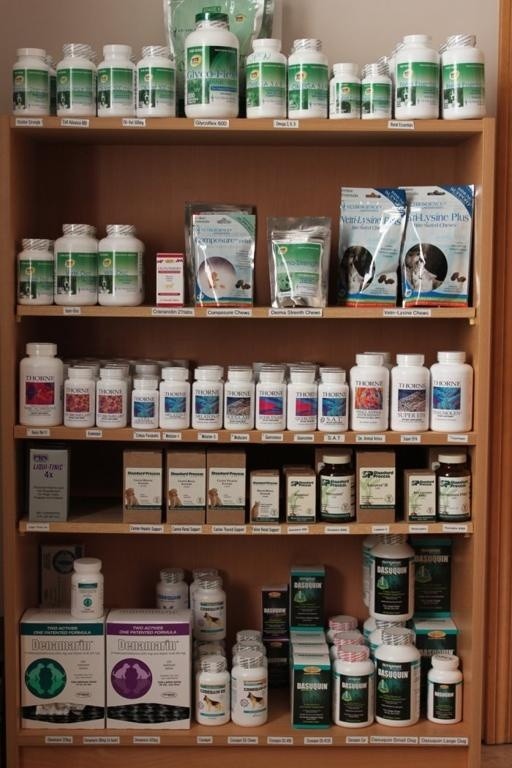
[339,248,365,294]
[406,243,443,292]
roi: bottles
[316,453,356,523]
[16,222,146,306]
[155,566,190,612]
[18,342,474,434]
[361,533,416,622]
[70,557,104,621]
[426,654,464,725]
[186,569,270,728]
[11,13,488,122]
[327,614,422,728]
[434,452,472,522]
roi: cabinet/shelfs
[0,113,496,768]
